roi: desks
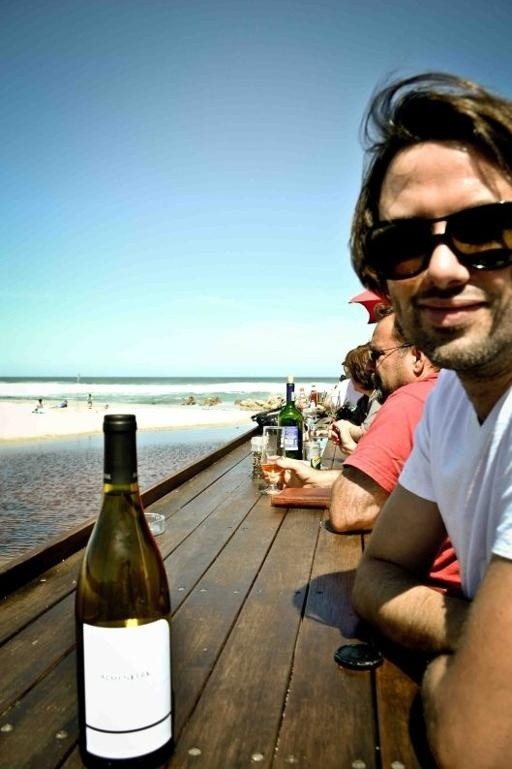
[0,424,439,769]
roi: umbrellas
[347,285,395,327]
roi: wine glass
[259,426,286,494]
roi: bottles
[311,384,317,407]
[73,411,177,762]
[278,375,304,461]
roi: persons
[87,394,95,409]
[32,398,45,414]
[51,399,68,409]
[342,71,511,769]
[254,301,464,593]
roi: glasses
[368,345,411,360]
[364,200,512,281]
